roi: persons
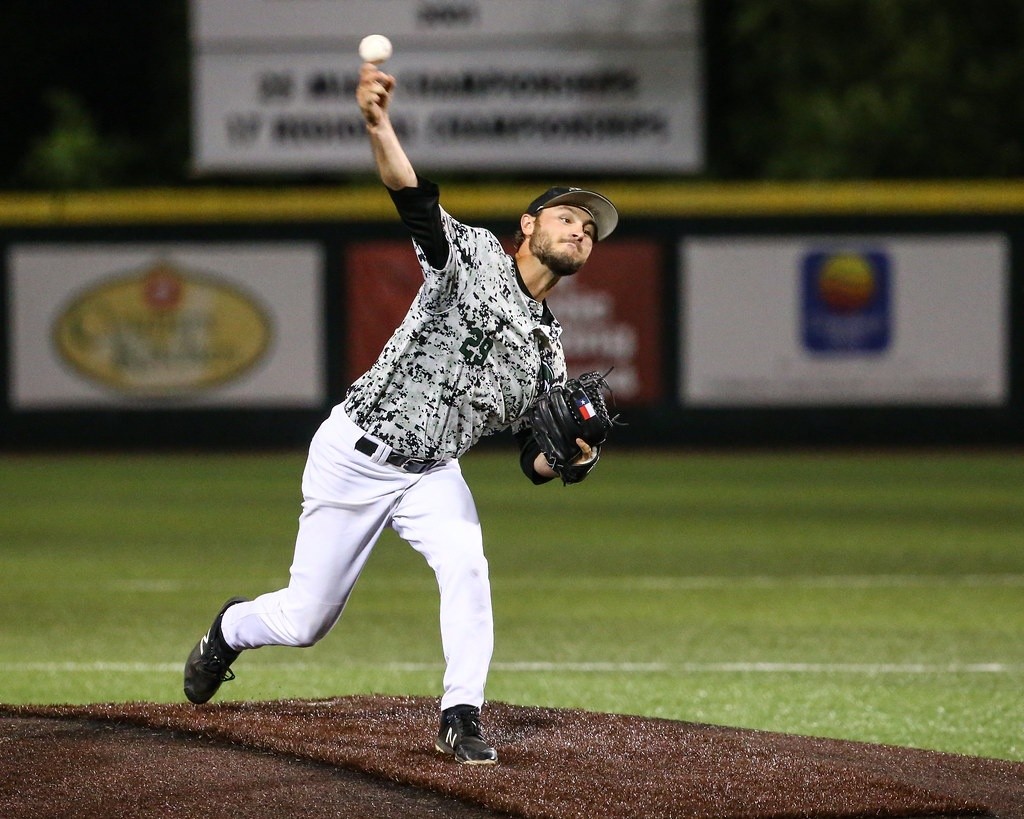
[184,60,629,765]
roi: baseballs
[358,34,393,63]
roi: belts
[355,436,446,474]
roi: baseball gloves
[500,364,631,486]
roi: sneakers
[435,705,498,765]
[184,596,248,704]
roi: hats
[526,187,618,240]
